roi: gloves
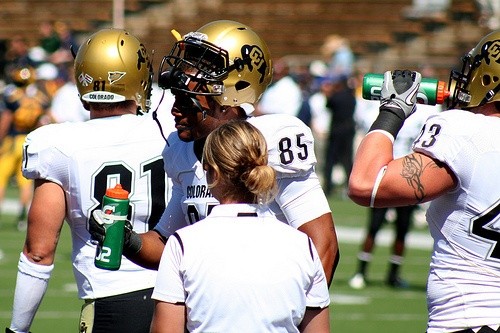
[88,209,143,259]
[365,69,420,142]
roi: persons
[0,29,92,231]
[6,28,178,333]
[88,20,341,290]
[150,120,331,333]
[349,28,500,333]
[253,33,362,195]
[349,94,422,289]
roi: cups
[93,183,131,270]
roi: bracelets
[367,104,405,144]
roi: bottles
[361,73,451,106]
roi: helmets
[454,29,500,109]
[70,28,154,113]
[182,20,273,107]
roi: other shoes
[384,277,404,287]
[349,273,366,289]
